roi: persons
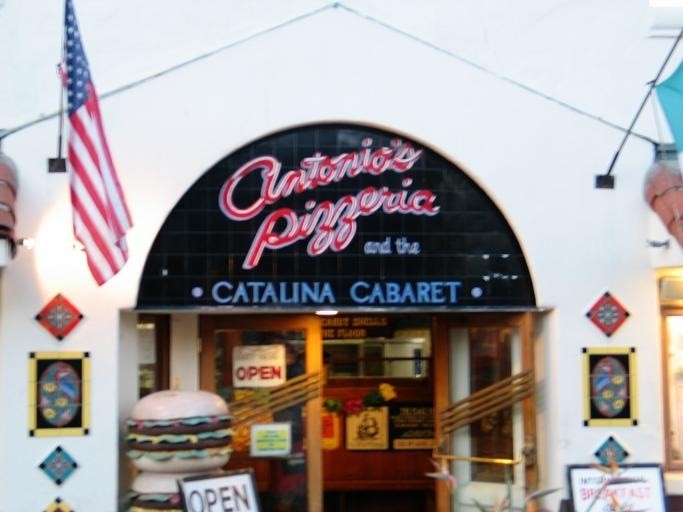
[266,342,303,506]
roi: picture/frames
[26,352,90,437]
[578,346,638,427]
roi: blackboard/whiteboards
[567,463,665,511]
[178,469,260,512]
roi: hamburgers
[118,388,239,473]
[127,468,227,511]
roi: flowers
[323,382,395,415]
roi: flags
[62,0,131,287]
